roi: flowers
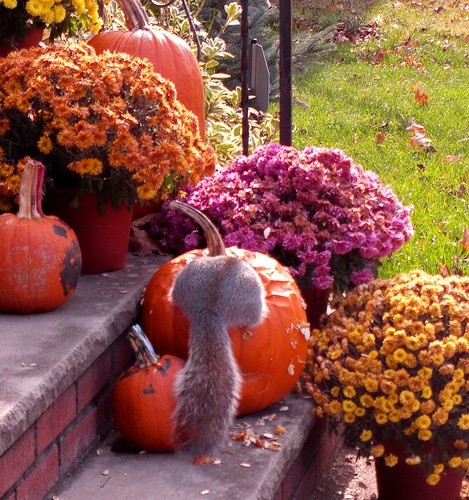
[0,44,216,215]
[295,270,469,487]
[150,143,414,290]
[0,0,111,45]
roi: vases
[299,285,331,331]
[373,437,465,500]
[47,187,133,274]
[0,27,45,58]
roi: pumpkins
[112,325,189,453]
[141,200,311,416]
[0,160,83,315]
[80,0,206,148]
